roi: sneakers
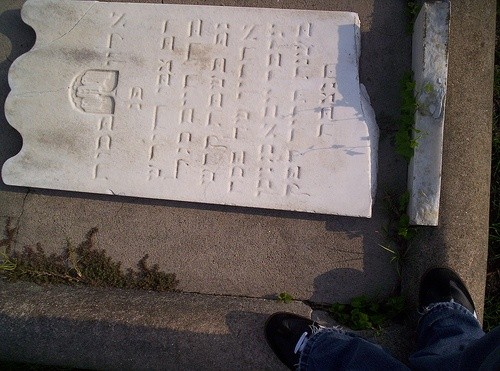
[264,310,330,368]
[418,264,481,327]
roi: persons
[264,264,500,371]
